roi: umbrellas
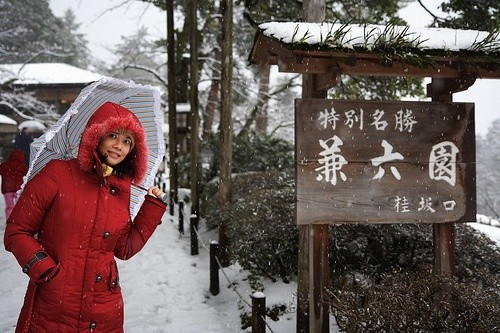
[18,122,46,131]
[12,79,167,222]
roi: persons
[16,127,34,164]
[3,101,168,333]
[0,150,29,221]
